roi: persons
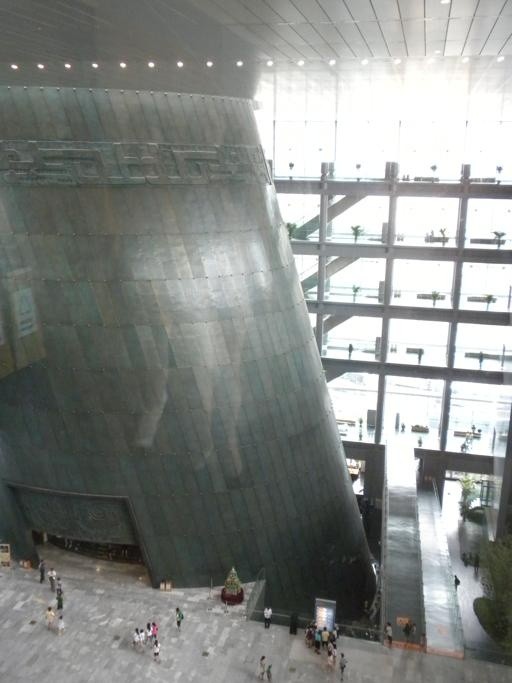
[38,559,65,636]
[411,623,417,635]
[385,621,392,645]
[474,553,480,574]
[304,621,348,681]
[132,621,160,663]
[455,575,460,591]
[258,655,267,680]
[263,606,273,627]
[266,663,273,681]
[175,608,184,629]
[403,621,412,639]
[419,633,425,647]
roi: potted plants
[222,565,243,605]
